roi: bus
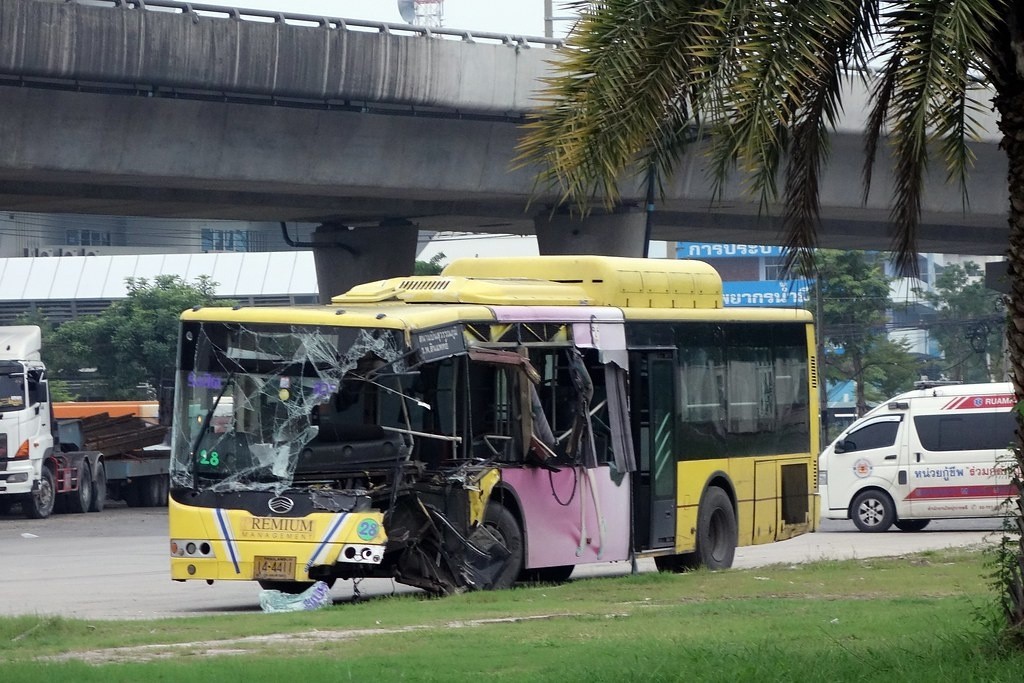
[159,253,821,602]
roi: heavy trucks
[0,325,171,519]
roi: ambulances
[816,379,1024,533]
